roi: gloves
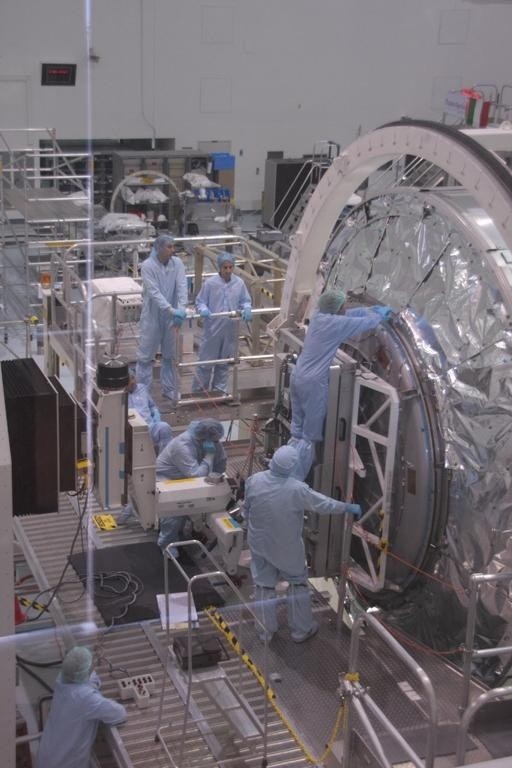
[173,310,186,326]
[203,441,214,451]
[370,306,392,321]
[242,308,252,321]
[344,503,362,517]
[200,309,208,317]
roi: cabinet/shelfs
[112,151,211,233]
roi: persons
[191,252,254,392]
[117,368,169,525]
[134,236,188,402]
[155,419,227,560]
[35,644,128,768]
[243,445,362,645]
[286,288,401,482]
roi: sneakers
[294,623,319,643]
[261,632,274,642]
